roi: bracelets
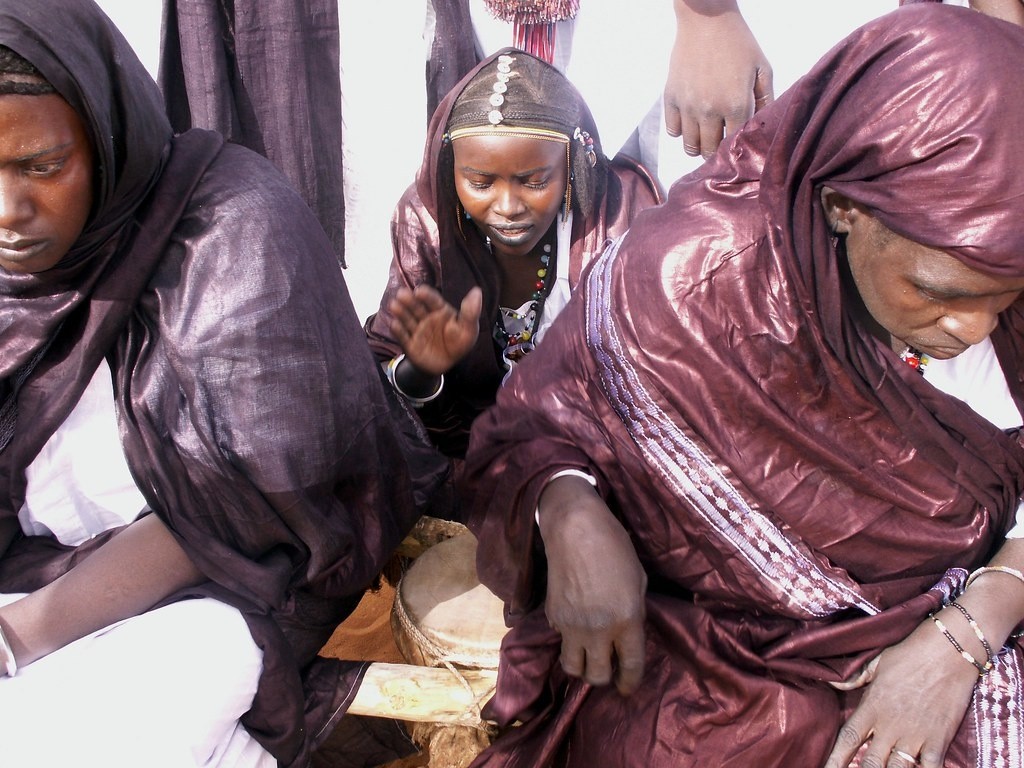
[533,467,599,526]
[944,602,994,673]
[391,352,447,403]
[0,625,19,678]
[387,353,440,408]
[927,611,987,677]
[961,566,1024,640]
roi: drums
[390,531,513,671]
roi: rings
[891,747,922,768]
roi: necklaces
[898,342,929,379]
[485,232,554,354]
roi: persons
[643,0,1022,267]
[444,0,1024,768]
[1,0,427,768]
[353,37,658,521]
